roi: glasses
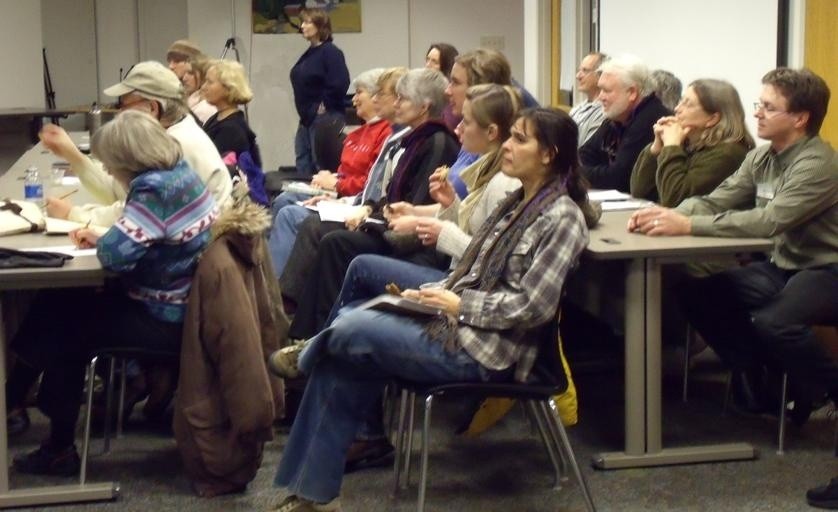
[754,101,790,119]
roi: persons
[626,66,835,437]
[568,52,757,454]
[4,39,262,471]
[261,43,591,512]
[265,103,592,512]
[284,5,352,175]
[805,471,837,509]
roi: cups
[420,282,445,291]
[49,169,65,186]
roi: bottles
[24,163,45,219]
[88,102,102,137]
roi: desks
[582,190,775,470]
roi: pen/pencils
[74,219,91,250]
[600,198,630,202]
[332,172,345,177]
[39,188,79,210]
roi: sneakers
[345,434,393,469]
[9,411,30,438]
[805,478,837,509]
[91,360,178,434]
[13,439,80,478]
[265,492,341,511]
[269,341,309,381]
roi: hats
[102,56,185,100]
[166,39,202,58]
